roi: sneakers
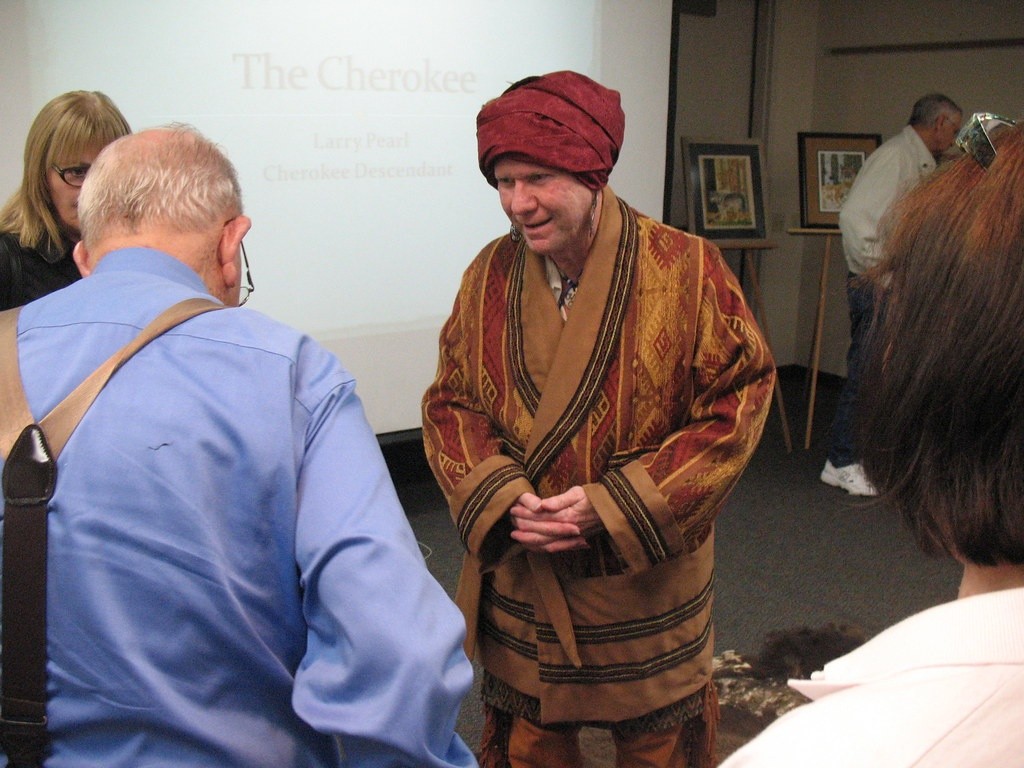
[821,460,877,495]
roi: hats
[475,72,626,193]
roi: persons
[0,90,132,311]
[715,115,1024,768]
[0,127,479,767]
[421,71,777,768]
[819,93,963,496]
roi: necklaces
[559,269,578,290]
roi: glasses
[955,113,1019,174]
[224,218,255,307]
[49,162,90,188]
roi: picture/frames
[680,138,773,238]
[798,132,883,230]
